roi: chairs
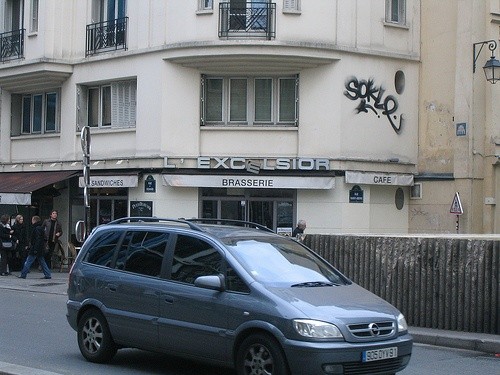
[56,239,77,273]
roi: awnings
[0,169,83,206]
[161,167,337,189]
[77,168,143,188]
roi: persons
[11,214,31,274]
[16,216,53,280]
[41,209,63,270]
[0,214,16,276]
[292,220,307,237]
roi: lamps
[472,39,500,84]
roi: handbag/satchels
[2,242,12,248]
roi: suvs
[65,216,413,375]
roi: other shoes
[41,276,51,279]
[0,273,8,276]
[15,274,26,279]
